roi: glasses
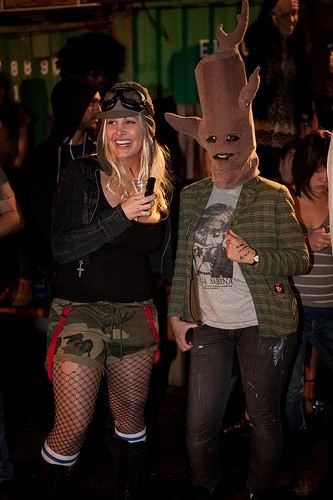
[100,87,150,115]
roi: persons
[285,129,333,468]
[0,32,128,306]
[39,82,174,500]
[239,0,315,180]
[304,0,333,132]
[1,165,25,493]
[273,134,303,187]
[167,114,310,500]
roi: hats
[96,80,155,175]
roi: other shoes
[289,443,318,470]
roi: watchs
[249,249,259,266]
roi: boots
[35,458,79,500]
[109,435,153,500]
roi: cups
[129,181,146,198]
